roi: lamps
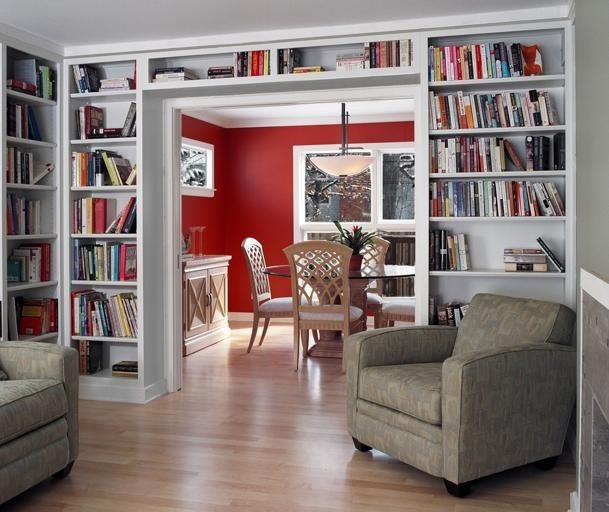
[310,103,375,178]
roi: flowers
[329,220,382,254]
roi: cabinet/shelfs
[141,30,419,99]
[181,254,233,357]
[415,18,578,326]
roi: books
[71,149,136,234]
[72,340,137,377]
[7,59,57,141]
[6,146,54,235]
[429,180,565,217]
[151,39,415,82]
[73,64,136,140]
[7,243,58,341]
[429,132,566,173]
[428,41,544,82]
[429,295,470,327]
[71,239,137,338]
[429,229,564,273]
[429,90,561,130]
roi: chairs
[240,232,414,373]
[0,337,79,507]
[344,292,576,498]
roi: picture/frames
[181,137,215,199]
